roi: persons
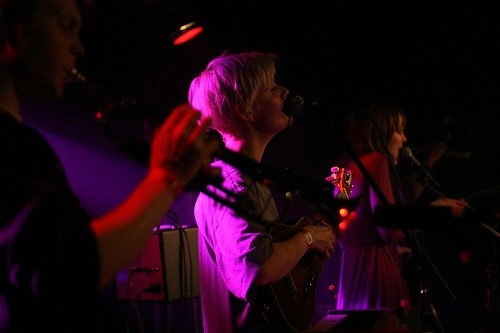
[323,98,477,320]
[185,52,336,333]
[0,1,220,333]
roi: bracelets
[300,229,314,247]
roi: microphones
[400,147,440,189]
[444,150,471,159]
[285,95,369,120]
